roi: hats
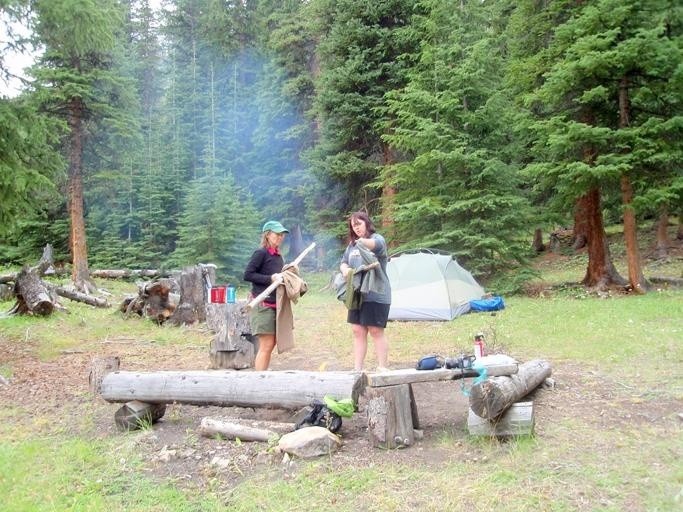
[263,221,289,234]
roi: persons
[339,209,392,371]
[242,218,309,372]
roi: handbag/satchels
[470,296,505,313]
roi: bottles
[210,286,219,303]
[217,285,225,303]
[226,283,235,303]
[473,335,485,358]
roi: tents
[384,247,488,323]
